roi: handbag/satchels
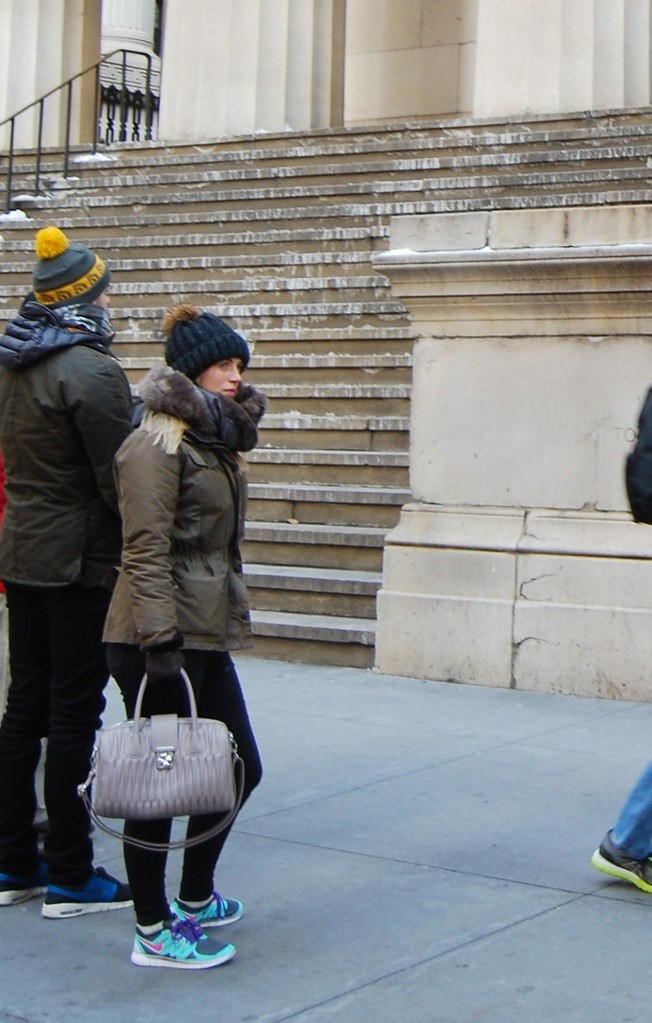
[78,667,245,851]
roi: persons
[1,225,137,919]
[99,302,268,972]
[586,381,652,897]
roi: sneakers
[0,864,48,905]
[592,829,652,893]
[131,913,237,969]
[169,891,243,927]
[41,866,134,918]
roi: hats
[33,227,112,310]
[161,302,250,382]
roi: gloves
[147,648,185,682]
